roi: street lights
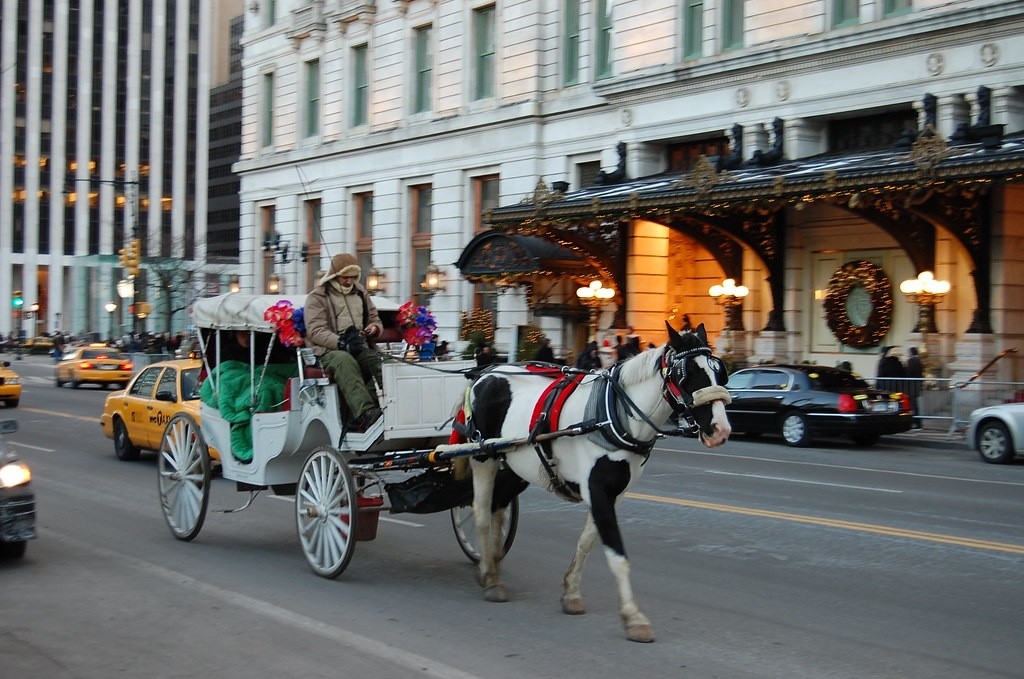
[710,277,750,355]
[576,280,614,351]
[901,271,952,389]
[31,302,39,341]
[104,301,117,343]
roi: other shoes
[910,426,923,433]
[359,408,381,431]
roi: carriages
[158,294,732,646]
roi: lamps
[229,275,240,292]
[365,264,387,295]
[267,272,282,294]
[422,264,448,292]
[972,123,1006,149]
[312,267,327,289]
[550,180,571,194]
[706,155,724,171]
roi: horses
[448,320,733,644]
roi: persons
[305,252,384,430]
[0,320,197,379]
[416,326,659,372]
[796,343,929,432]
[229,331,250,365]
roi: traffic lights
[119,248,127,267]
[128,238,139,277]
[128,304,134,312]
[12,292,23,308]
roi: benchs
[296,327,409,386]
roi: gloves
[339,327,366,353]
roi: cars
[0,359,23,409]
[1,337,51,356]
[174,337,204,360]
[53,343,135,387]
[96,358,224,466]
[670,362,914,444]
[1,419,39,564]
[965,398,1024,464]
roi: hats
[317,253,362,286]
